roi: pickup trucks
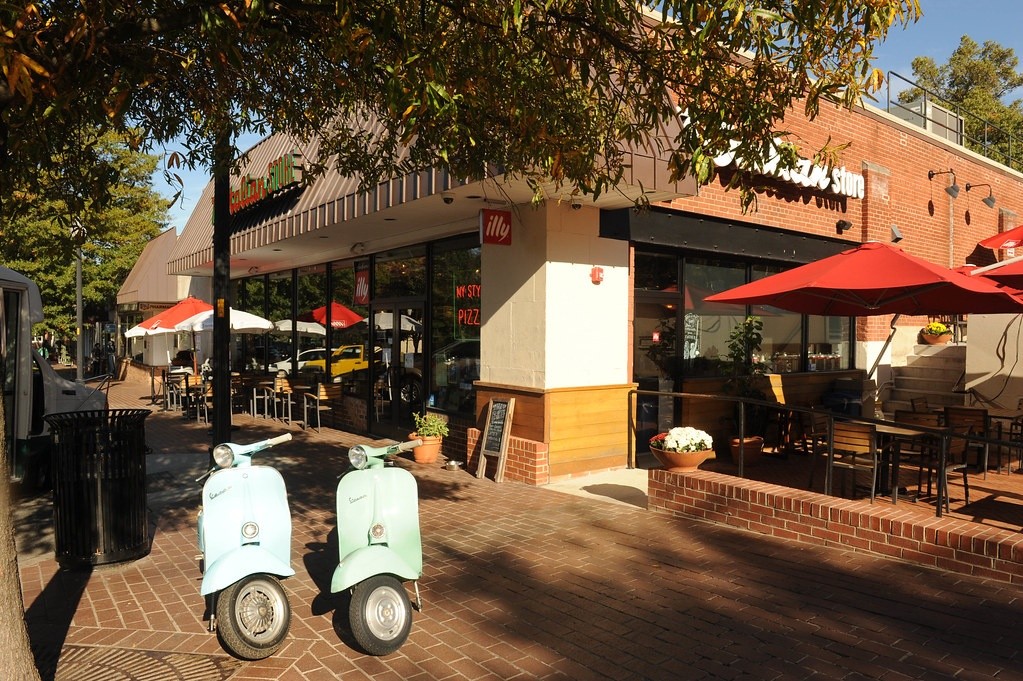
[301,345,384,378]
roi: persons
[124,295,275,423]
[42,334,51,365]
[91,343,101,382]
[107,341,115,378]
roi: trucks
[0,265,119,494]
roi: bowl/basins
[445,460,464,470]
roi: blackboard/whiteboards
[481,397,515,457]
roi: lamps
[967,182,995,210]
[928,170,960,198]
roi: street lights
[71,215,86,381]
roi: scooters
[331,438,423,657]
[195,434,295,660]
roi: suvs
[388,338,480,405]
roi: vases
[919,331,955,347]
[649,446,715,473]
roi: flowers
[650,427,714,452]
[919,320,954,334]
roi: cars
[262,348,359,376]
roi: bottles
[752,350,843,374]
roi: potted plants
[716,314,773,466]
[408,411,447,464]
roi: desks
[292,385,317,426]
[257,381,274,418]
[931,405,1023,476]
[841,418,945,497]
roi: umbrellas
[363,311,423,332]
[298,299,364,330]
[657,283,782,317]
[269,320,326,361]
[702,223,1023,316]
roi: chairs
[808,397,1023,512]
[164,376,344,433]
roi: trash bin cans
[43,408,153,568]
